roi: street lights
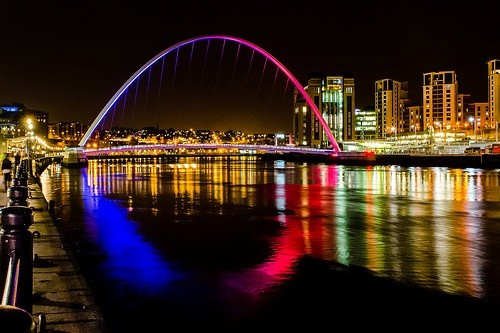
[389,121,467,145]
[470,117,481,139]
[26,118,35,157]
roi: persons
[2,156,12,191]
[14,152,21,176]
